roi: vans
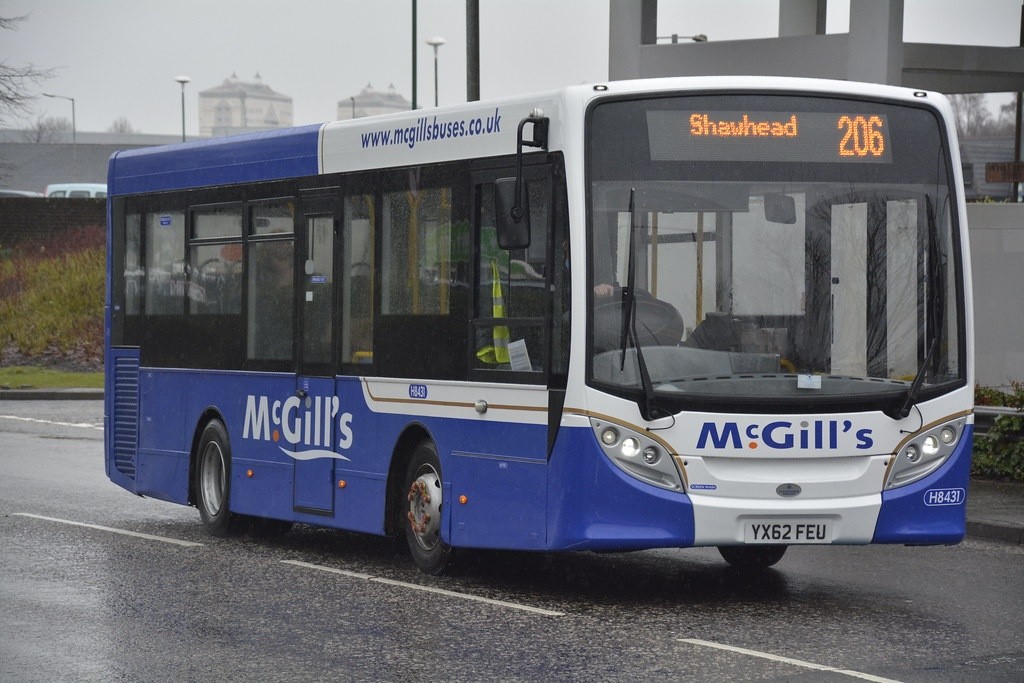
[45,182,108,199]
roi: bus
[99,74,980,583]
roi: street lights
[426,37,444,109]
[174,75,191,144]
[40,91,78,179]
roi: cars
[0,190,38,199]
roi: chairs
[151,258,467,318]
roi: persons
[201,229,330,361]
[551,229,627,372]
